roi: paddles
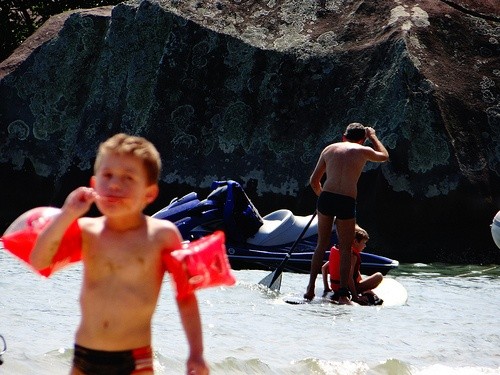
[257,199,319,300]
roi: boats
[146,178,399,278]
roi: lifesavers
[2,204,82,279]
[163,230,235,298]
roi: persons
[321,223,383,306]
[303,122,389,304]
[30,132,210,375]
